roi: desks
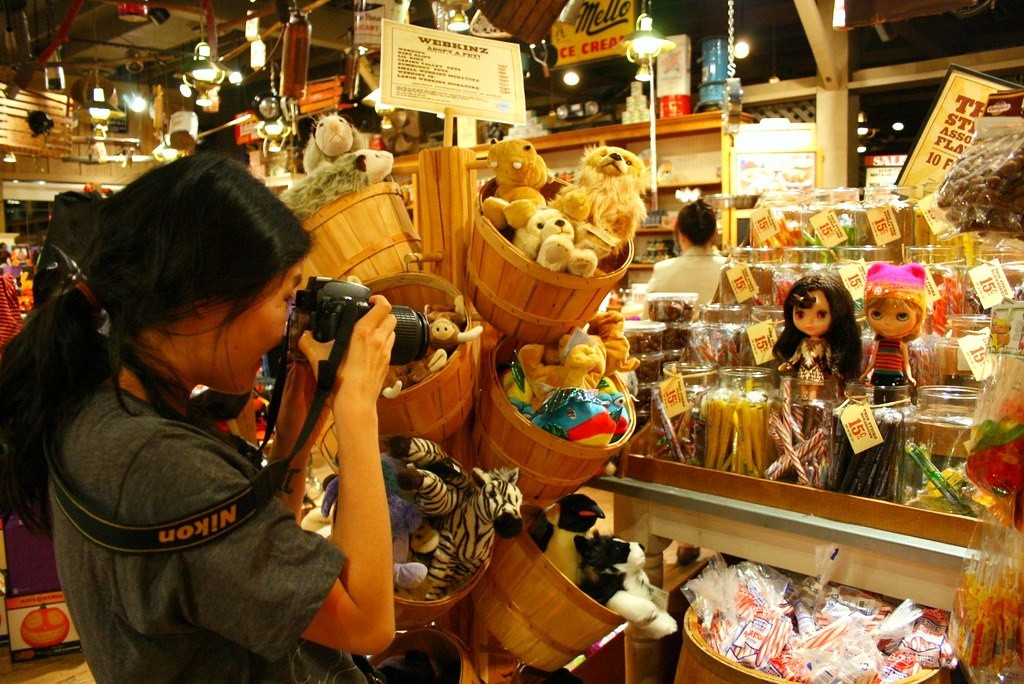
[589,476,1023,684]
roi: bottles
[898,384,982,517]
[772,374,839,489]
[702,365,772,477]
[45,61,66,91]
[280,8,311,98]
[651,361,716,466]
[344,50,361,93]
[690,185,1024,383]
[828,378,916,503]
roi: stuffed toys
[279,112,524,601]
[480,138,678,638]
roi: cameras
[295,276,429,365]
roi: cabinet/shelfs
[389,110,757,302]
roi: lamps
[1,0,677,175]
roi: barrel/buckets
[466,175,635,342]
[674,601,950,684]
[367,629,480,684]
[395,557,491,630]
[291,181,424,297]
[473,525,621,673]
[474,338,636,501]
[365,270,481,447]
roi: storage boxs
[656,34,691,98]
[0,520,84,665]
[659,96,693,116]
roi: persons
[859,262,928,404]
[646,198,727,305]
[772,273,862,400]
[0,152,396,684]
